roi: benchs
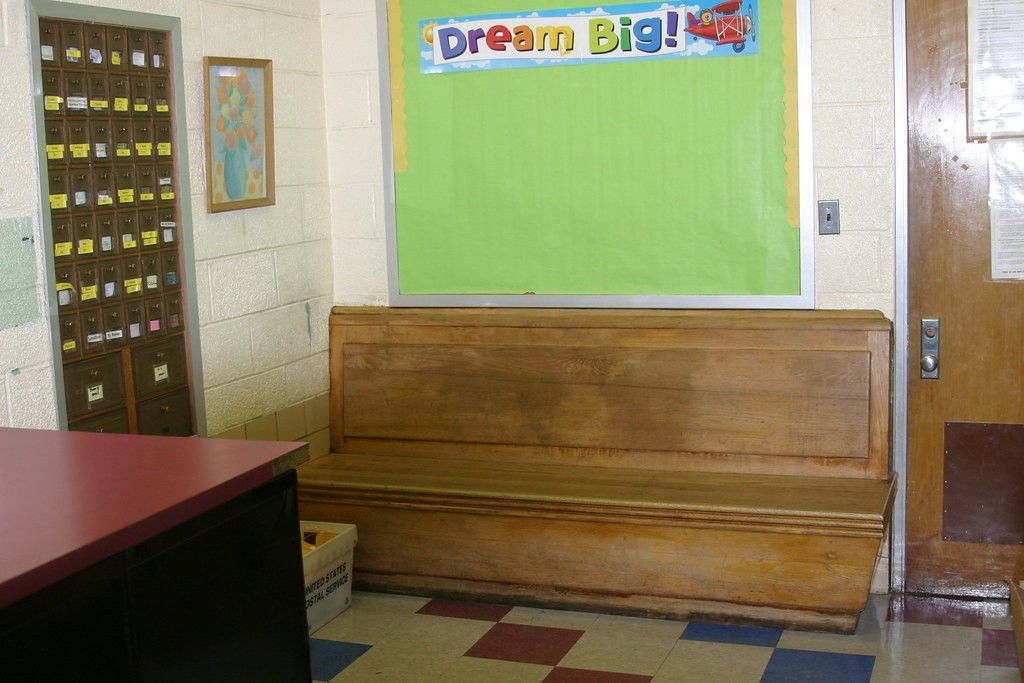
[297,306,899,634]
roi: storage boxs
[300,520,357,635]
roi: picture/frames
[203,56,276,213]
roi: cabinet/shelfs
[39,15,196,436]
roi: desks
[0,424,313,682]
